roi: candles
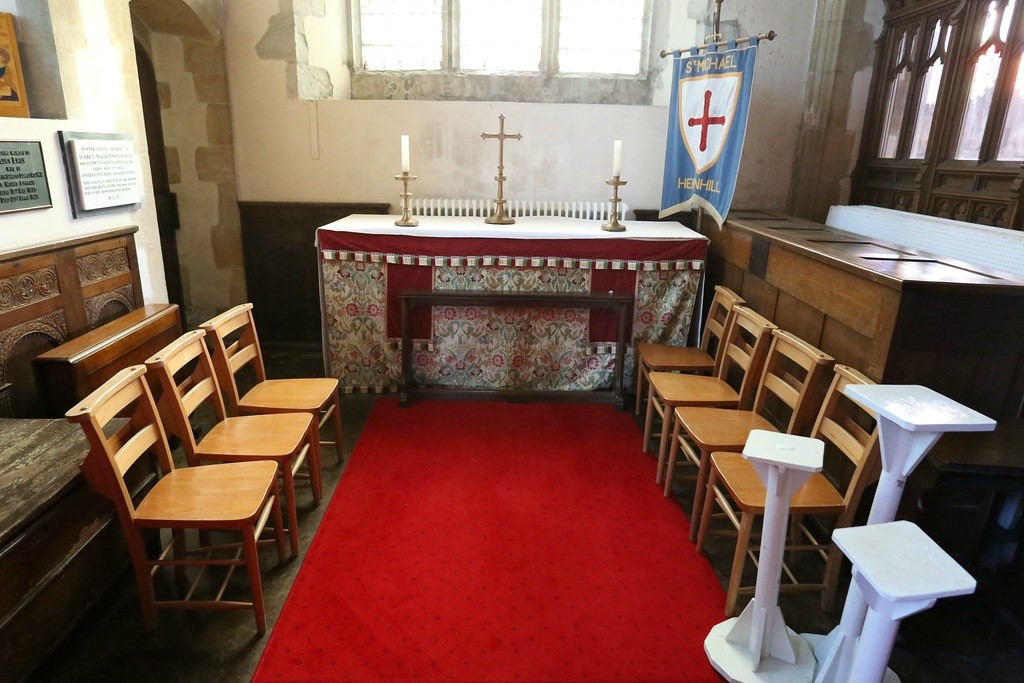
[401,135,409,172]
[613,140,622,177]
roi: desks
[315,213,710,394]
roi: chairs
[643,305,778,484]
[696,364,881,618]
[635,284,745,424]
[145,329,320,557]
[199,303,344,499]
[664,328,836,541]
[65,365,287,635]
